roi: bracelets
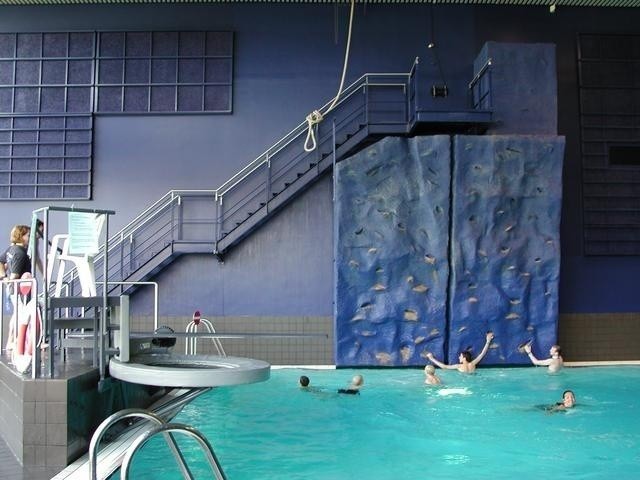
[1,274,8,279]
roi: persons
[0,223,32,355]
[535,389,578,414]
[352,374,363,388]
[423,365,441,385]
[298,374,309,388]
[426,332,494,374]
[524,344,565,373]
[6,218,51,351]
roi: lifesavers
[14,302,40,372]
